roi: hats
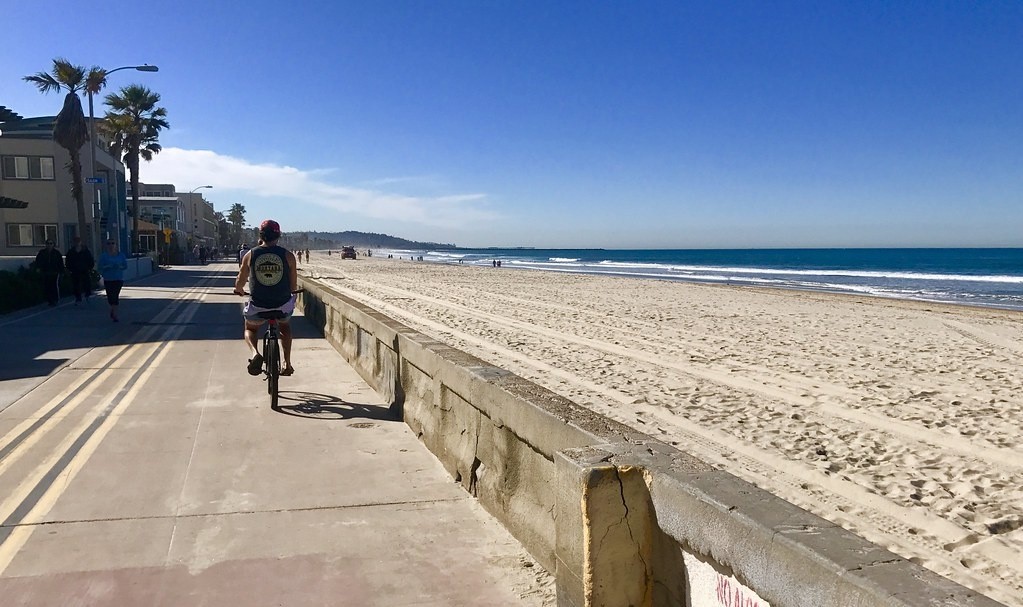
[261,220,280,234]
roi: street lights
[190,186,213,263]
[89,64,159,276]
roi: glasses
[106,242,116,245]
[46,242,54,245]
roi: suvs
[341,246,356,259]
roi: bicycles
[234,288,306,409]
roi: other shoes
[282,361,294,376]
[110,308,119,323]
[248,354,263,376]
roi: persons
[363,249,371,256]
[298,250,302,263]
[306,248,310,264]
[234,220,298,376]
[388,254,392,259]
[291,247,296,254]
[492,259,502,267]
[411,256,423,261]
[214,248,217,254]
[36,238,65,307]
[66,237,94,306]
[237,243,250,261]
[96,239,128,321]
[192,244,210,263]
